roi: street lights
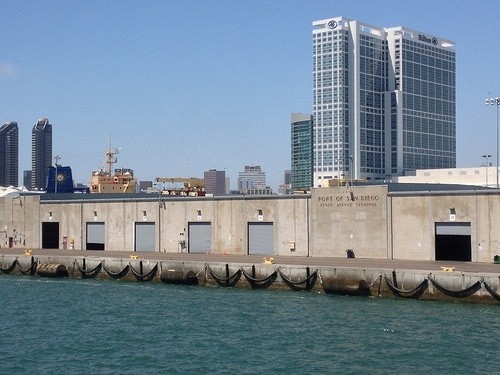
[54,155,61,193]
[349,156,353,186]
[484,97,500,189]
[481,154,492,187]
